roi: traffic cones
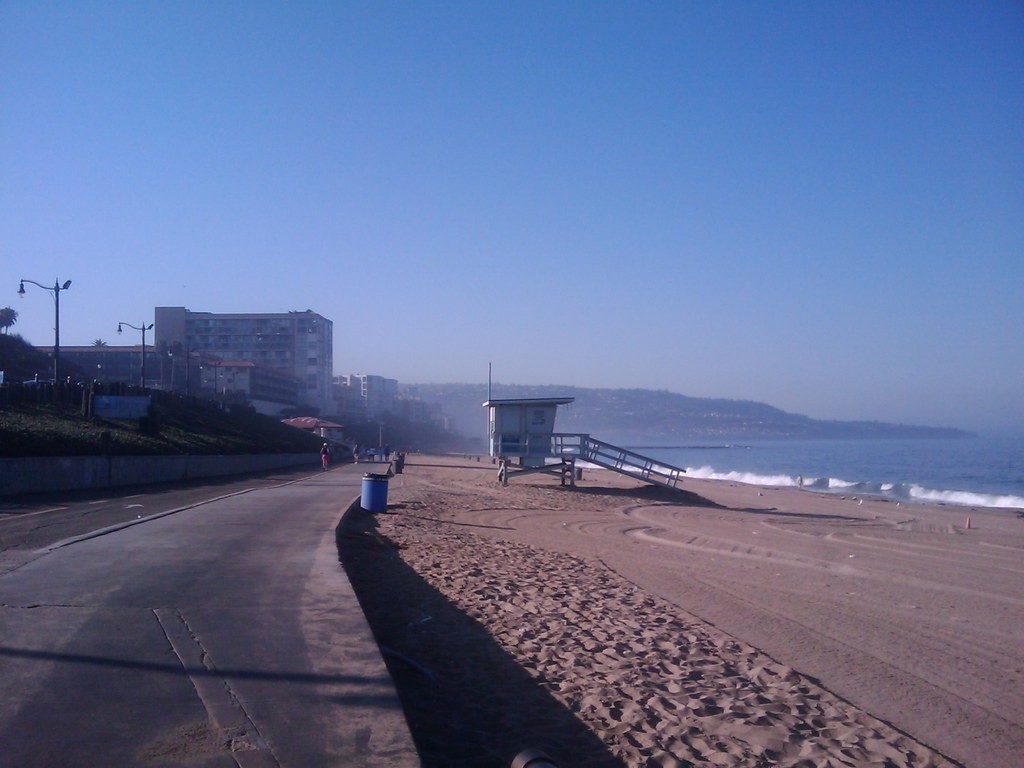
[966,517,972,529]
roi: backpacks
[322,447,327,454]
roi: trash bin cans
[396,455,404,468]
[359,472,389,514]
[392,460,403,474]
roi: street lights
[17,276,72,384]
[167,345,189,395]
[117,320,154,390]
[199,362,218,395]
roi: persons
[320,443,330,471]
[368,447,375,461]
[353,444,359,464]
[384,444,390,461]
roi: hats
[324,443,327,446]
